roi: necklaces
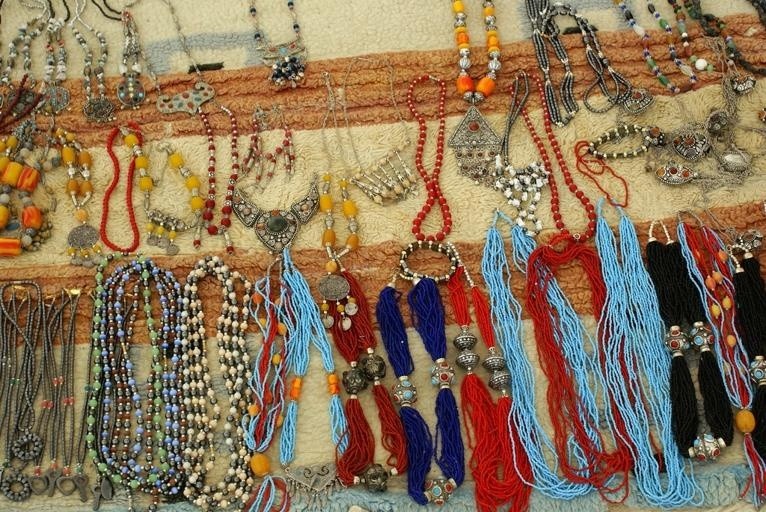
[1,0,763,512]
